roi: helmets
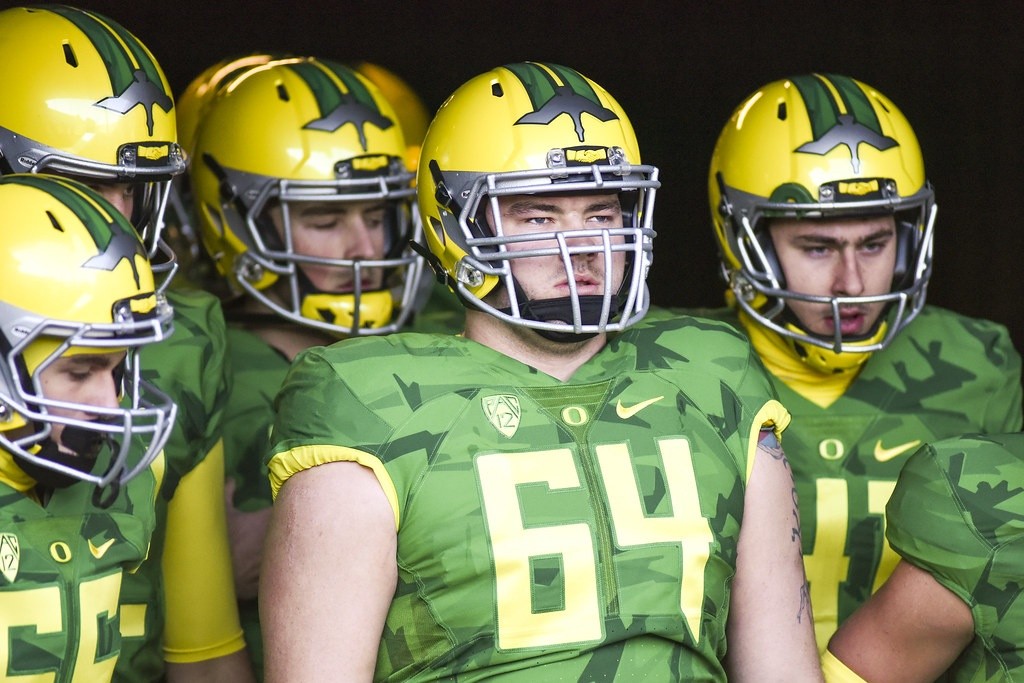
[709,72,937,373]
[1,2,186,182]
[416,60,642,342]
[173,52,427,335]
[0,173,157,488]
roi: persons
[0,1,1024,683]
